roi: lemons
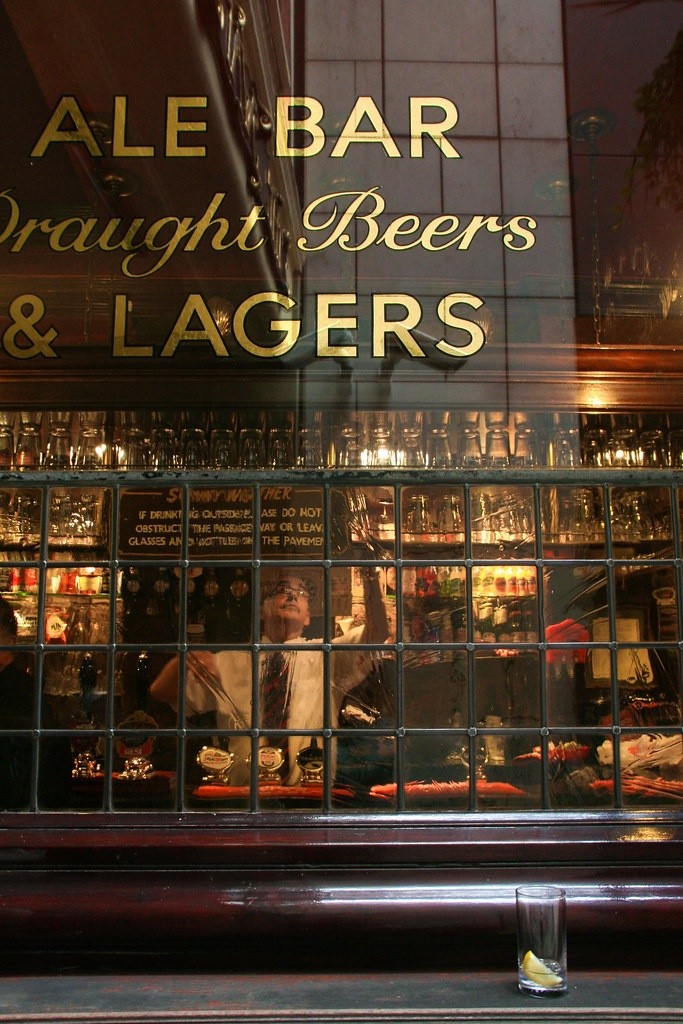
[523,951,562,985]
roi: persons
[0,597,67,814]
[150,566,390,786]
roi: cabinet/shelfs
[0,369,683,661]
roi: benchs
[0,813,683,1024]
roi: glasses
[262,584,310,597]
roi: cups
[514,883,568,999]
[0,409,683,470]
[407,495,465,544]
[470,494,536,542]
[542,487,673,541]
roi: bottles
[401,565,539,651]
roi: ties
[262,650,290,786]
